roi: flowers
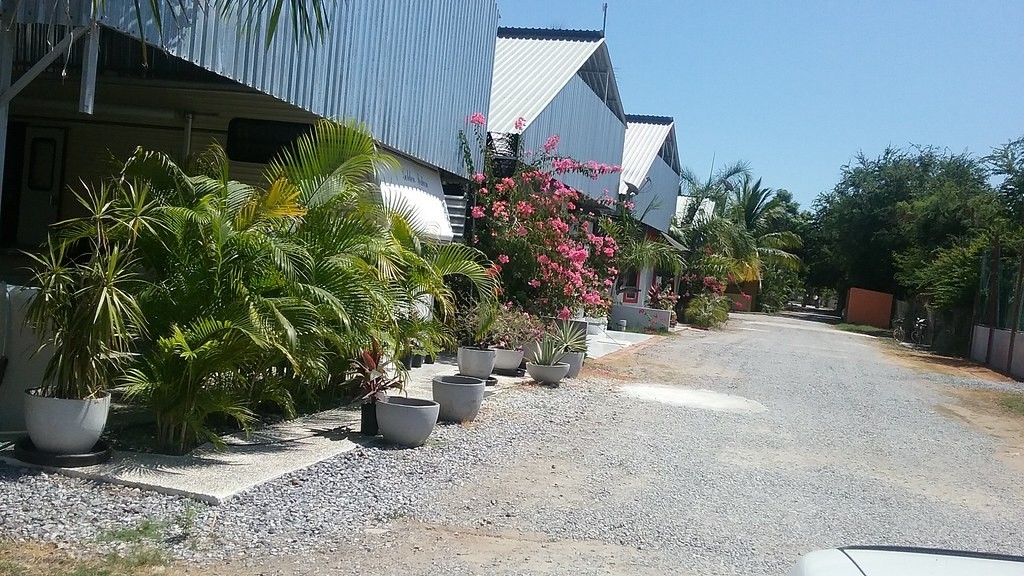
[484,303,541,353]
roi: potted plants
[15,174,175,466]
[544,317,591,380]
[340,337,406,436]
[516,330,570,384]
[392,336,438,370]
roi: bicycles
[911,317,927,345]
[892,315,905,343]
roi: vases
[432,373,486,424]
[454,345,499,386]
[487,345,526,377]
[376,395,440,448]
